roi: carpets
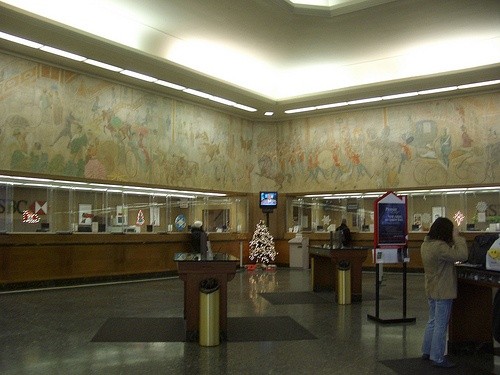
[90,316,318,342]
[378,354,493,375]
[259,291,395,304]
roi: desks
[308,246,372,301]
[448,266,500,355]
[174,252,240,341]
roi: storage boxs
[244,264,277,271]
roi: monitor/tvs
[260,191,277,207]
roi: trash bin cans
[199,279,219,347]
[337,261,352,303]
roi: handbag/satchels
[468,235,498,265]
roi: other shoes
[422,354,430,360]
[433,357,458,367]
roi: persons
[420,215,470,367]
[191,220,211,254]
[335,218,352,247]
